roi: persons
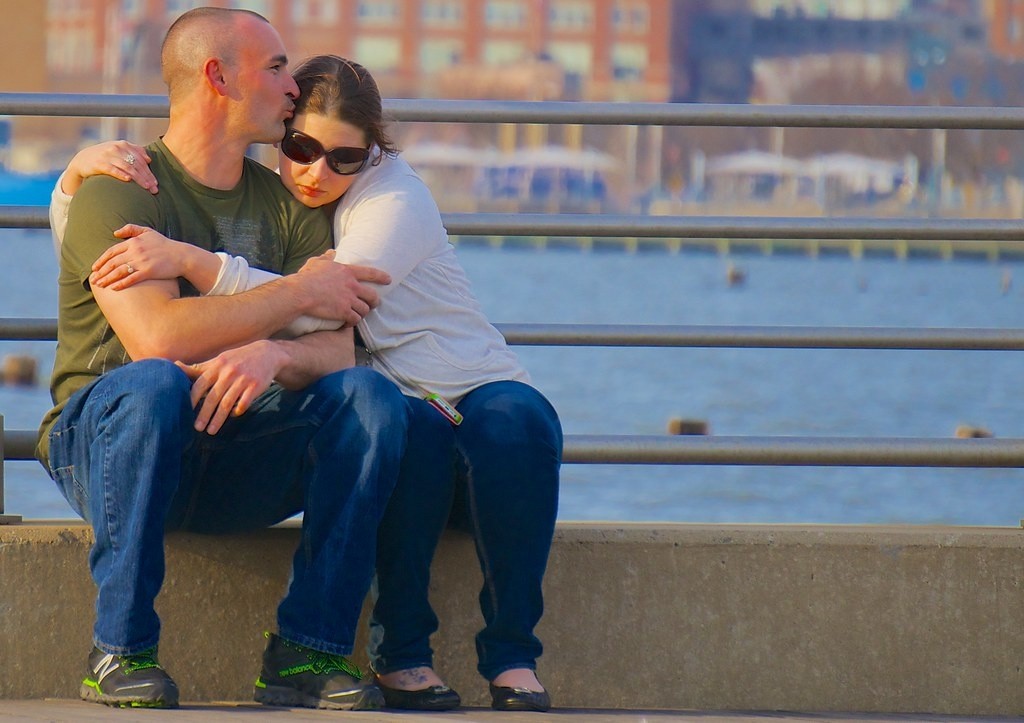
[48,52,564,712]
[32,6,392,711]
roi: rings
[124,155,136,165]
[125,264,133,273]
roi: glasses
[280,126,372,176]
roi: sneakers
[79,645,180,708]
[252,635,386,709]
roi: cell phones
[424,394,461,424]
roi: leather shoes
[489,669,551,711]
[373,670,462,711]
